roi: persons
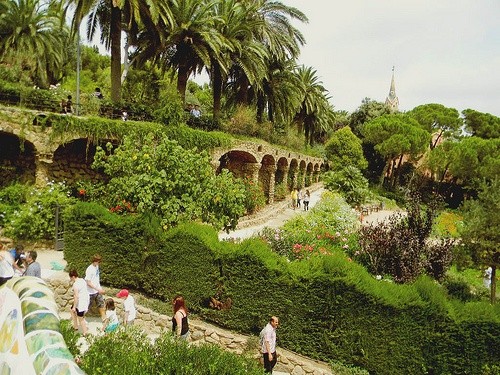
[69,270,90,337]
[10,245,25,274]
[303,190,310,211]
[185,104,192,112]
[116,289,135,325]
[25,251,41,278]
[263,317,278,374]
[291,188,298,210]
[0,244,14,285]
[297,189,302,208]
[171,297,190,340]
[85,255,106,321]
[190,105,200,118]
[65,95,74,113]
[101,298,118,334]
[94,88,102,98]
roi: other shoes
[102,316,106,323]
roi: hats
[116,289,128,298]
